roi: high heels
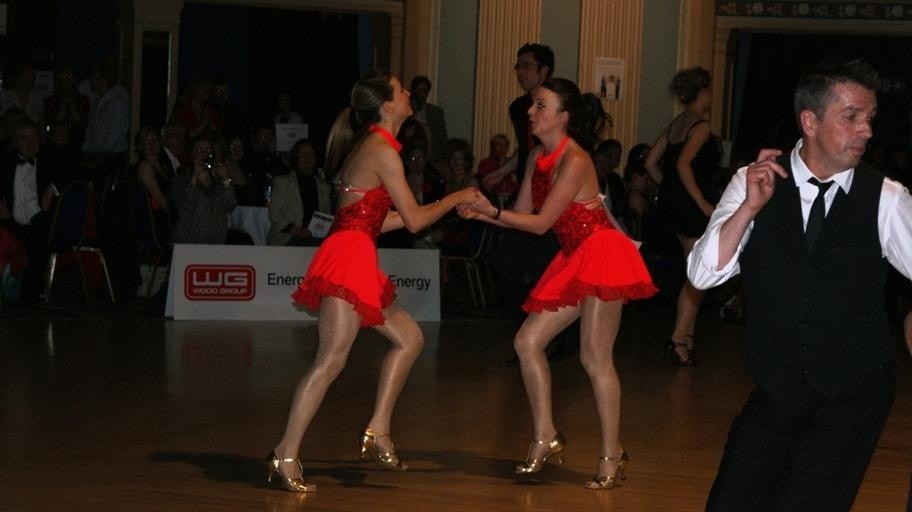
[264,446,318,493]
[357,425,410,473]
[583,445,631,490]
[514,430,566,475]
[662,332,697,369]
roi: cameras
[198,152,217,171]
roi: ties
[805,176,835,246]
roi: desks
[227,206,271,246]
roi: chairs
[440,221,487,310]
[18,178,169,315]
